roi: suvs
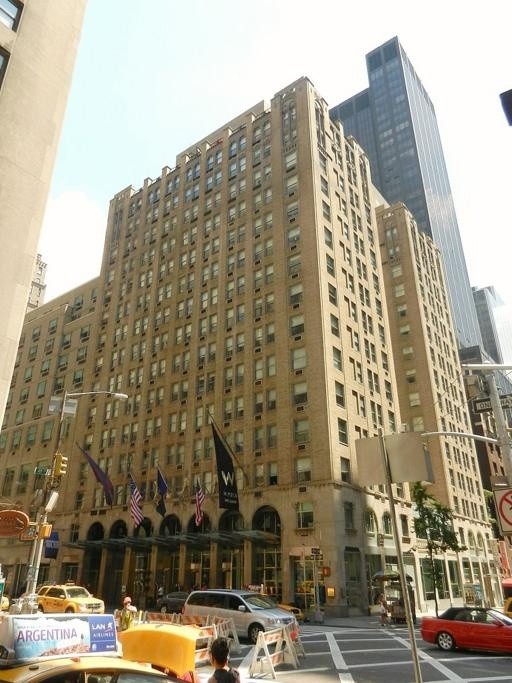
[182,589,298,644]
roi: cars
[0,610,196,683]
[156,591,191,615]
[262,594,305,621]
[421,597,512,655]
[38,583,106,614]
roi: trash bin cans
[314,607,325,623]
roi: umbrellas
[371,569,412,581]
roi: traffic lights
[54,454,68,477]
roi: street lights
[26,391,128,594]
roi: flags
[79,447,115,507]
[128,474,144,529]
[210,420,239,511]
[44,530,61,559]
[155,468,167,518]
[195,477,207,526]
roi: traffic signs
[473,393,512,413]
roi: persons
[377,592,389,627]
[118,596,137,630]
[150,582,207,602]
[205,636,241,682]
